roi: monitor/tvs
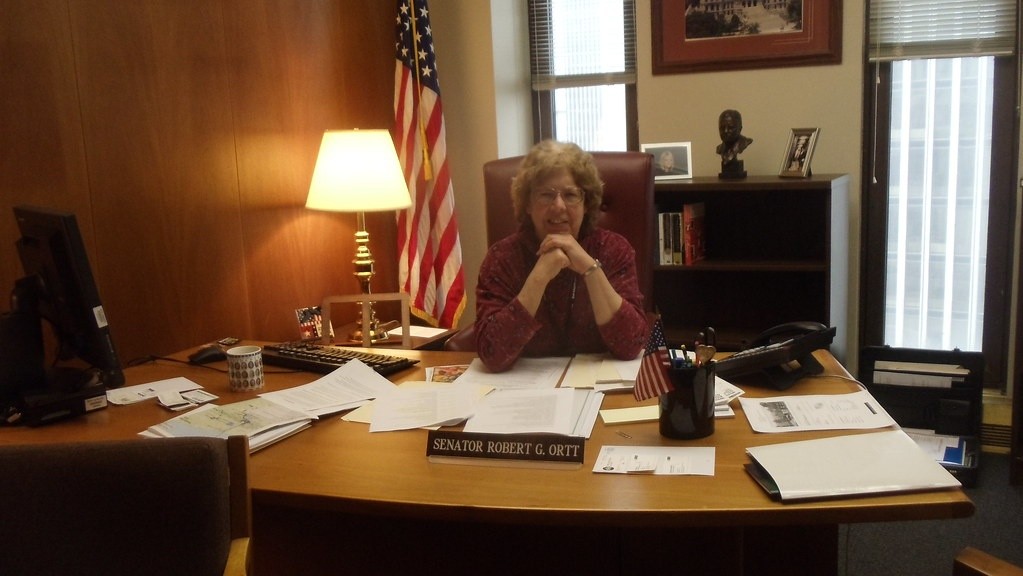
[12,203,126,397]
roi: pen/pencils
[672,349,677,359]
[695,341,699,364]
[707,327,717,347]
[699,332,705,344]
[681,345,688,360]
[682,362,692,368]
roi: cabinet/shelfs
[655,173,850,369]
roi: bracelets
[584,258,602,279]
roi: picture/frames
[778,127,821,178]
[641,142,693,180]
[651,0,843,75]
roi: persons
[789,144,806,171]
[659,151,679,175]
[476,141,651,373]
[716,109,753,155]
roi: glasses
[533,186,586,206]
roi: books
[658,200,706,265]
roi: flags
[633,319,675,402]
[391,0,468,330]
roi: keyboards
[261,342,421,376]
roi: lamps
[305,130,412,342]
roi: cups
[226,346,265,393]
[658,359,717,439]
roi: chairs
[0,435,256,576]
[482,151,654,314]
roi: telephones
[714,321,837,391]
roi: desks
[0,337,977,576]
[308,318,461,350]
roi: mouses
[188,343,230,363]
